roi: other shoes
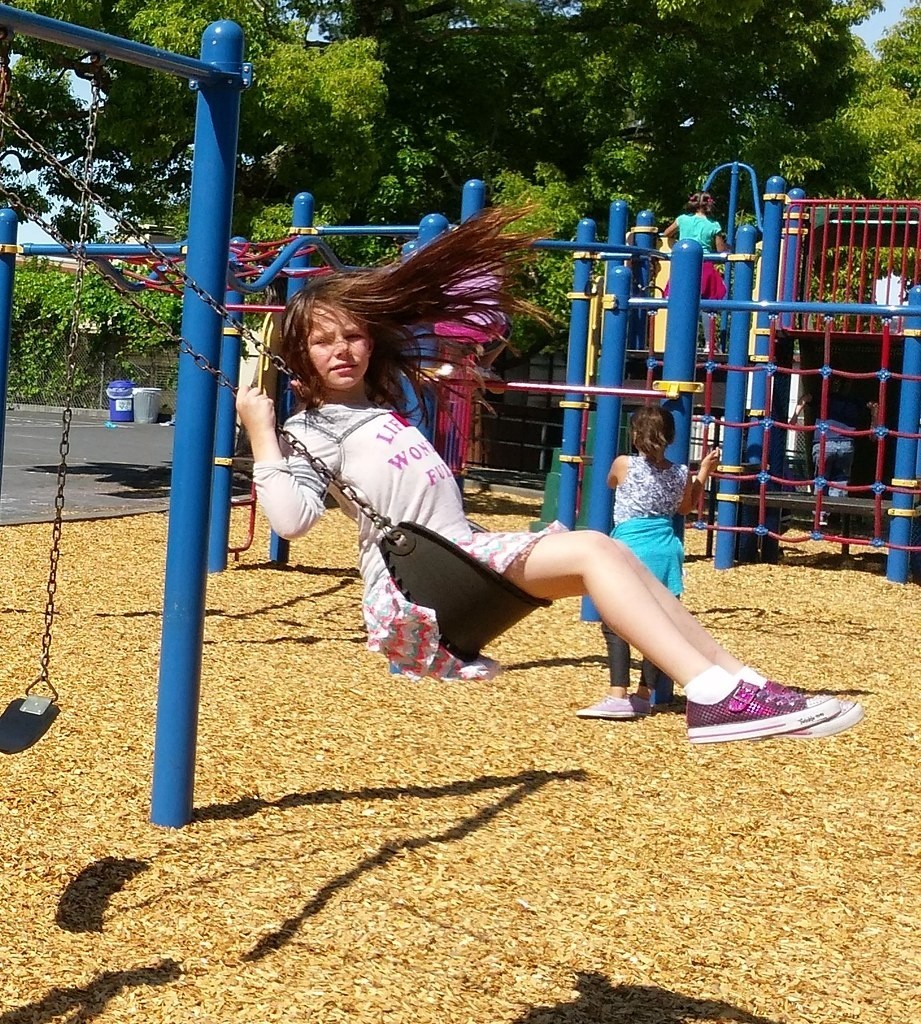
[818,512,828,525]
[703,341,719,353]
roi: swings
[0,27,110,756]
[0,104,555,665]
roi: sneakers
[576,694,635,719]
[686,679,840,745]
[629,693,652,718]
[749,680,864,741]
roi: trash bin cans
[107,380,137,421]
[132,388,163,423]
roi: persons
[662,192,732,353]
[434,225,513,394]
[576,405,720,720]
[235,207,863,743]
[787,380,879,533]
